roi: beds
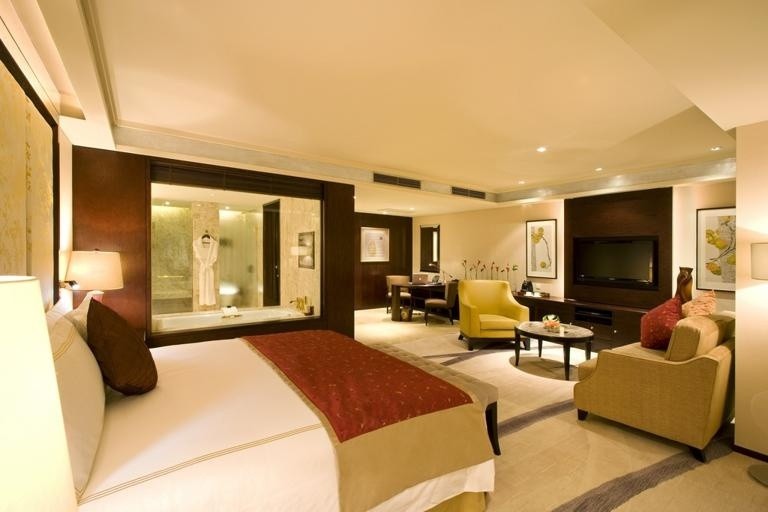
[43,288,500,512]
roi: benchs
[366,342,501,457]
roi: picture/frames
[298,232,314,270]
[526,219,557,280]
[696,206,736,293]
[361,227,390,262]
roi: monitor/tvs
[571,236,659,290]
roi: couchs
[573,335,735,464]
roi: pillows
[43,289,159,503]
[639,289,735,362]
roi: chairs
[424,282,458,325]
[385,275,414,313]
[459,280,530,352]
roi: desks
[391,283,445,321]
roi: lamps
[1,273,81,512]
[748,242,768,487]
[65,251,124,303]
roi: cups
[525,292,531,296]
[533,292,540,296]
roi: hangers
[202,231,211,245]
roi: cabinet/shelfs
[513,295,648,353]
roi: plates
[533,296,542,297]
[524,295,533,296]
